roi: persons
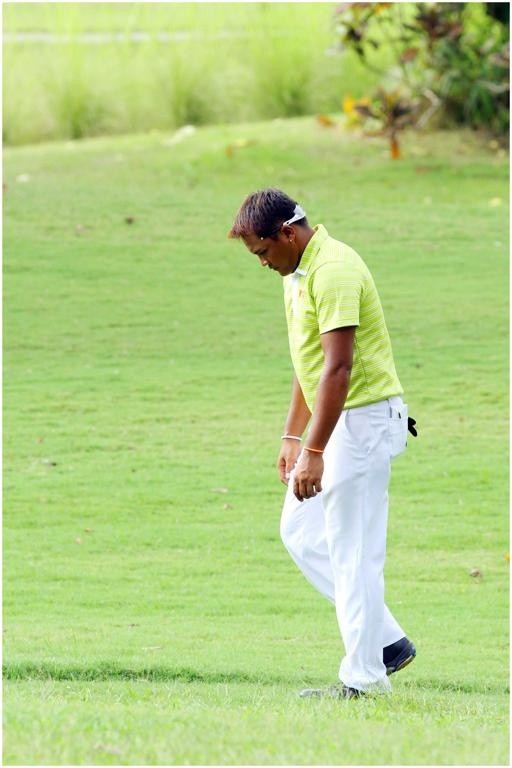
[223,186,418,698]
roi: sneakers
[299,682,364,701]
[382,638,415,676]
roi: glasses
[260,204,306,240]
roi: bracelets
[280,434,304,439]
[302,446,324,455]
